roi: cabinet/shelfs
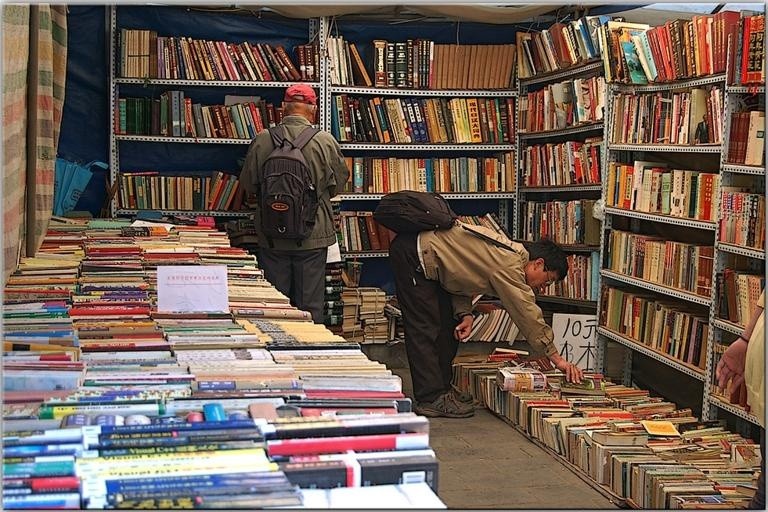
[515,46,767,449]
[108,5,514,258]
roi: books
[726,110,765,167]
[1,324,447,511]
[458,212,511,240]
[530,408,761,509]
[448,347,692,435]
[537,250,599,300]
[457,302,527,345]
[607,230,713,297]
[607,161,719,223]
[515,16,605,78]
[339,150,515,194]
[115,27,319,82]
[331,95,515,145]
[520,137,602,186]
[3,217,312,323]
[607,87,723,146]
[519,78,607,133]
[598,10,766,86]
[120,163,247,212]
[716,265,764,328]
[522,200,602,245]
[328,35,516,90]
[720,183,766,250]
[118,91,284,140]
[599,281,709,372]
[325,261,405,344]
[339,210,394,251]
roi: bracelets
[739,335,749,343]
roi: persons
[389,223,584,418]
[716,289,767,507]
[239,85,350,324]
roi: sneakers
[448,385,473,404]
[414,392,475,417]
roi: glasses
[544,264,553,285]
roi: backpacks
[260,126,322,248]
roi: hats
[284,85,316,104]
